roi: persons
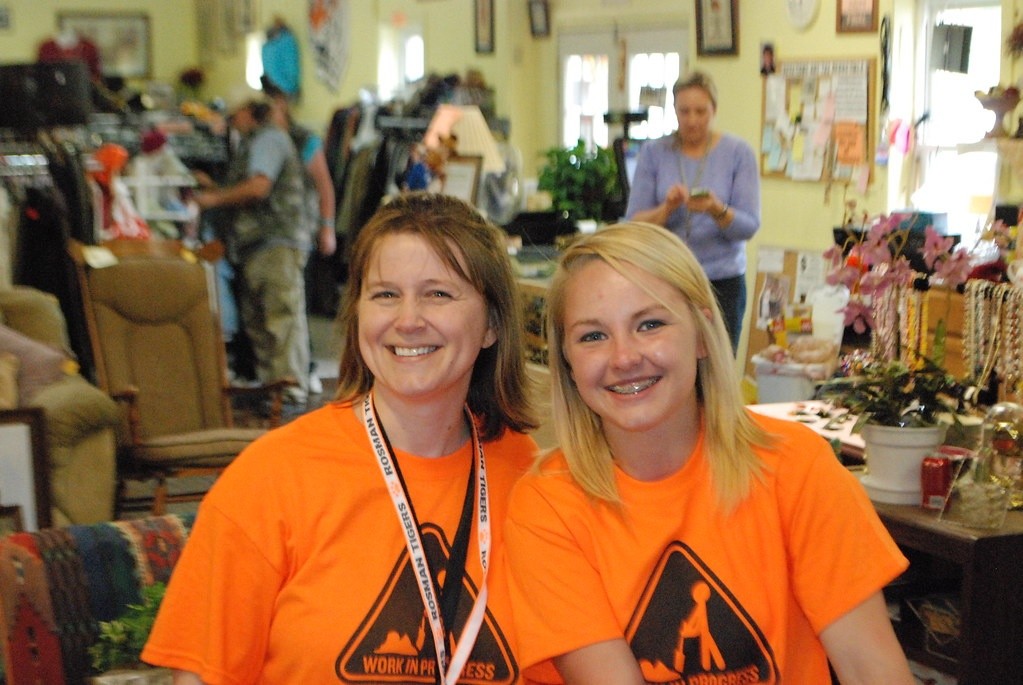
[624,69,760,364]
[177,73,338,425]
[139,189,543,684]
[760,43,776,75]
[501,221,929,685]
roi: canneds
[920,453,953,514]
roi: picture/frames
[474,0,495,52]
[695,0,742,57]
[0,406,50,533]
[527,0,550,37]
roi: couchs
[0,284,122,525]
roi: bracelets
[714,202,730,220]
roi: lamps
[448,106,507,173]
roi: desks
[746,397,1023,685]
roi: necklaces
[371,393,477,685]
[860,252,1022,389]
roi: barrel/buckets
[752,348,828,404]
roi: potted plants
[814,317,981,505]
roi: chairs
[63,232,299,517]
[0,514,190,685]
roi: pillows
[0,322,67,410]
